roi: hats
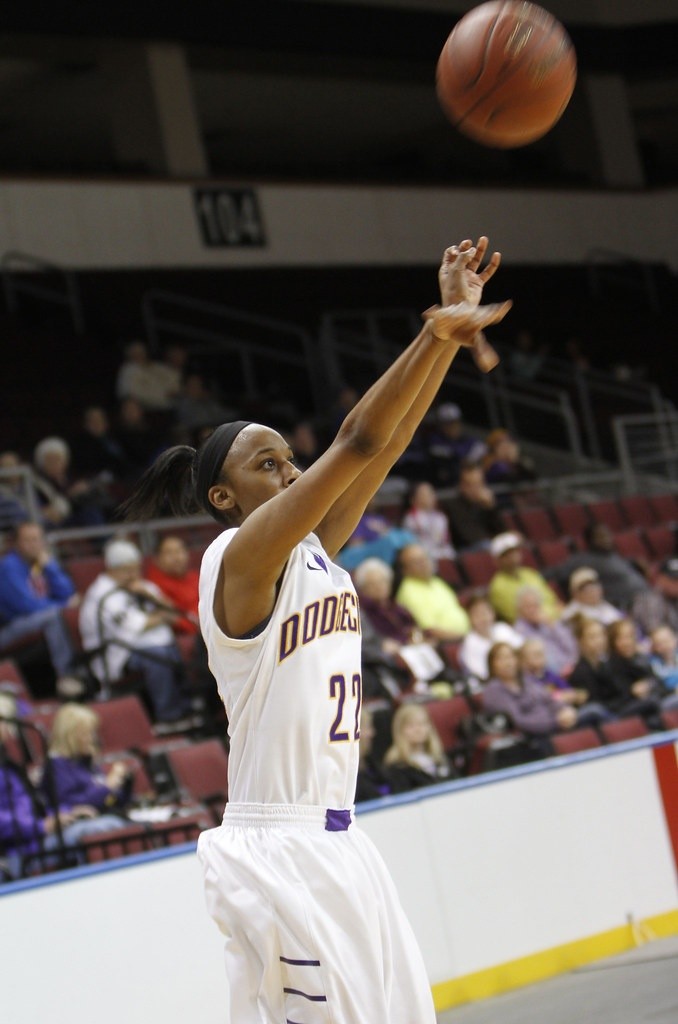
[104,541,141,570]
[488,530,520,557]
[436,401,462,422]
[569,568,599,592]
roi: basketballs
[435,1,579,149]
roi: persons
[0,335,678,863]
[117,235,515,1024]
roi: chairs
[0,496,678,864]
